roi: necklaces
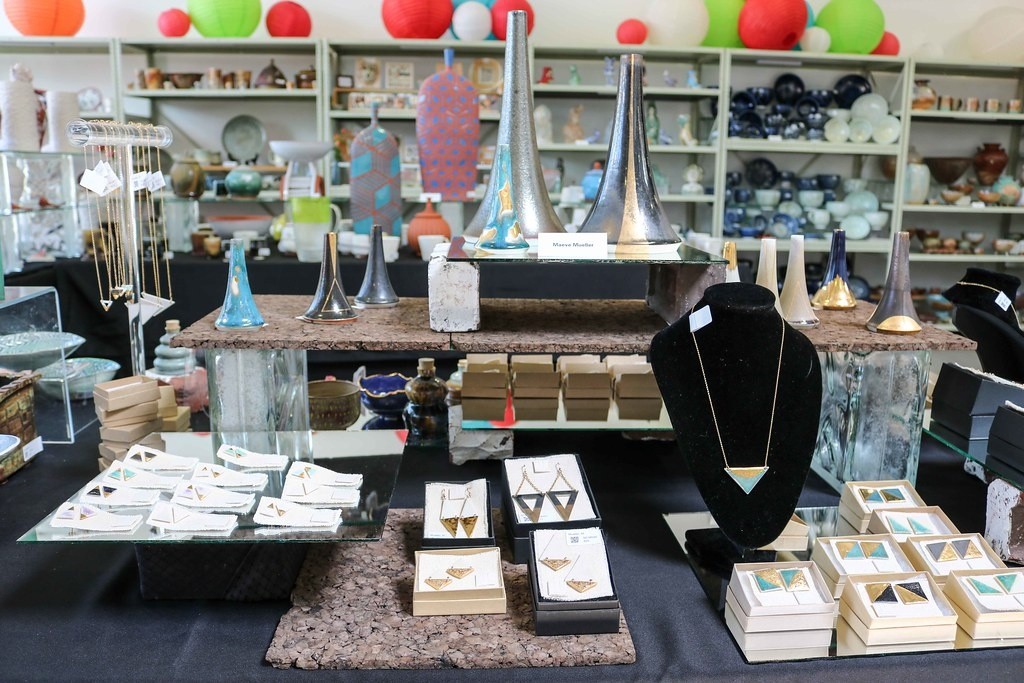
[83,119,176,326]
[956,280,1020,329]
[690,301,786,496]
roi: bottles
[402,358,450,438]
[912,79,937,110]
[974,143,1008,186]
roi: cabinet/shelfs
[322,42,531,200]
[118,37,323,203]
[896,57,1024,263]
[0,148,115,271]
[531,44,729,240]
[0,35,115,118]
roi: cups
[131,68,252,88]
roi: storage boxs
[410,355,1024,663]
[92,373,191,471]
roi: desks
[720,48,910,252]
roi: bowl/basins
[723,73,1024,321]
[308,380,360,430]
[34,357,121,400]
[165,73,204,88]
[357,373,415,414]
[0,332,86,372]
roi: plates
[221,115,267,161]
